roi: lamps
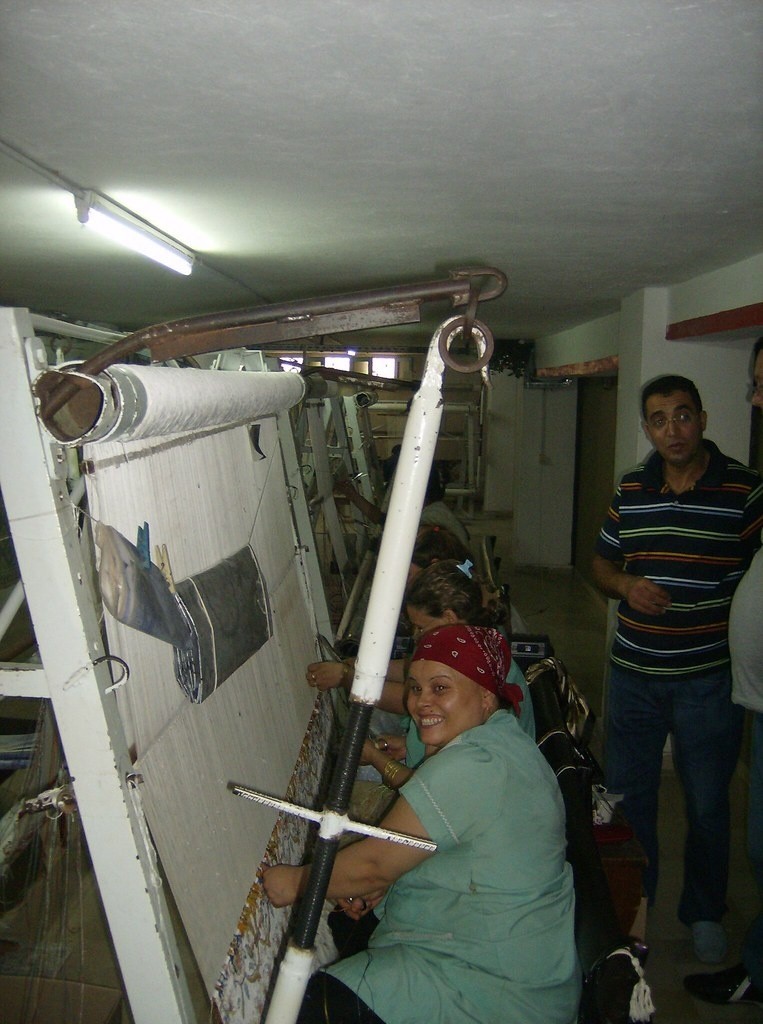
[76,190,195,276]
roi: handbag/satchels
[524,657,590,741]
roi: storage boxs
[611,889,648,941]
[511,633,554,673]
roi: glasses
[411,618,435,633]
[647,411,699,427]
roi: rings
[312,673,316,680]
[314,681,317,686]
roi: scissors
[344,897,369,912]
[333,471,368,496]
[367,729,388,751]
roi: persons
[259,444,582,1024]
[588,375,763,963]
[682,337,763,1004]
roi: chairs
[524,657,656,1024]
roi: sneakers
[683,962,763,1008]
[691,920,727,964]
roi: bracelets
[382,759,404,788]
